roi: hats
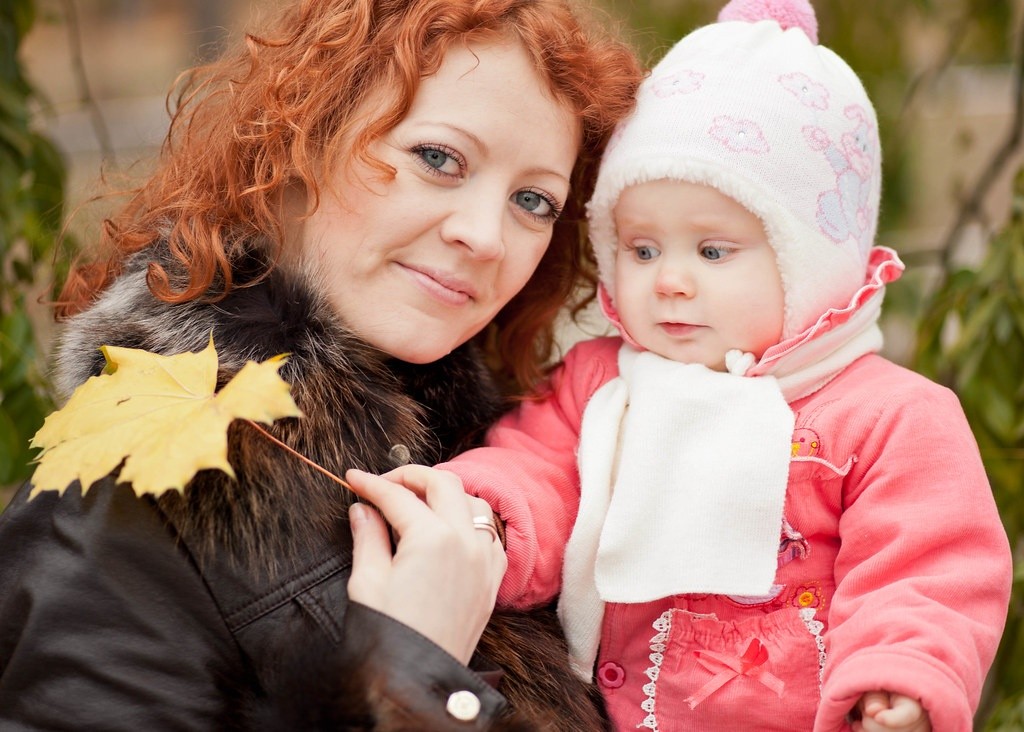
[587,3,881,341]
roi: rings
[471,515,497,540]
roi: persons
[428,0,1015,732]
[2,0,656,732]
